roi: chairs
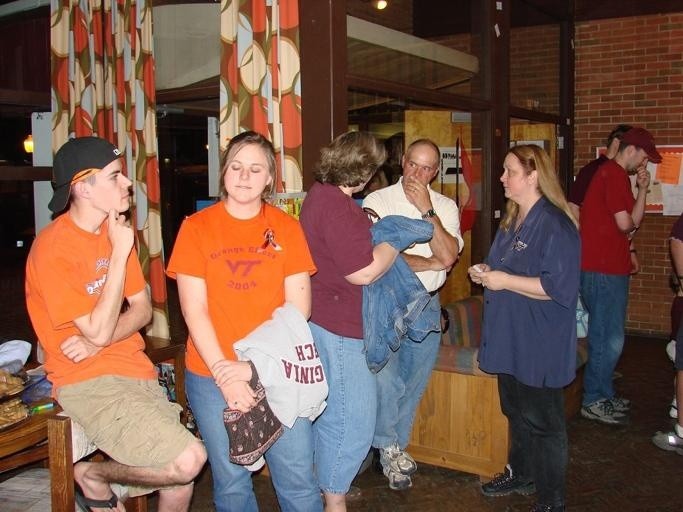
[0,406,150,512]
[405,292,590,485]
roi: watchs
[421,209,435,219]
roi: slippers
[74,479,118,512]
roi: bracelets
[630,250,636,252]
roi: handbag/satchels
[222,361,284,465]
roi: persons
[565,125,639,379]
[164,132,325,512]
[468,145,580,511]
[362,138,465,490]
[25,135,209,511]
[580,127,663,425]
[296,129,433,512]
[650,210,683,458]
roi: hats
[48,136,121,213]
[610,125,663,163]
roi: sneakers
[380,443,417,490]
[530,502,564,511]
[581,397,632,426]
[652,431,683,456]
[666,404,678,418]
[480,464,538,496]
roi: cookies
[0,369,28,426]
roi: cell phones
[473,265,482,273]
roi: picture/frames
[595,145,682,215]
